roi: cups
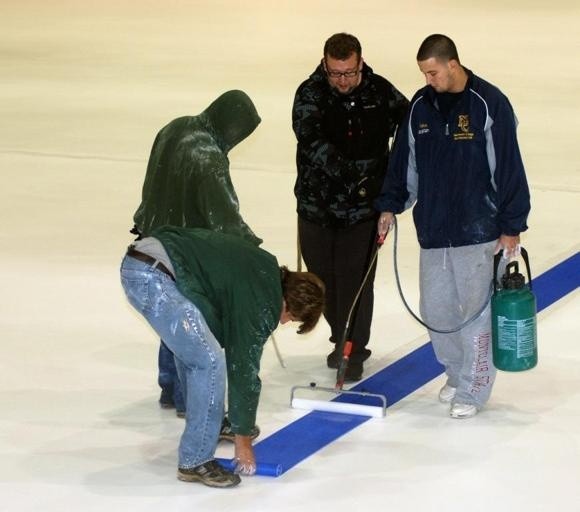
[324,59,359,78]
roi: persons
[287,28,411,387]
[127,89,264,420]
[375,32,532,421]
[119,221,328,490]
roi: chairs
[217,418,261,445]
[336,353,364,382]
[451,399,480,419]
[176,461,242,489]
[327,347,370,367]
[438,382,458,403]
[158,391,186,418]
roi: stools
[126,244,178,286]
